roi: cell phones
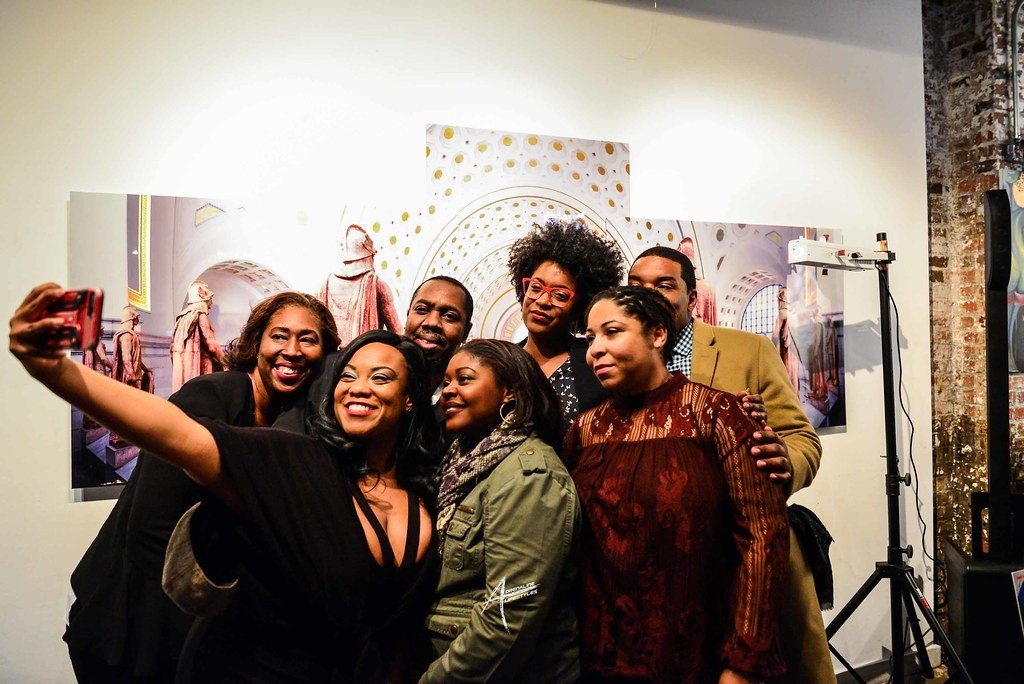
[32,287,104,350]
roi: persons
[402,274,474,514]
[822,314,841,386]
[82,319,112,430]
[312,224,405,350]
[629,246,838,684]
[803,308,826,400]
[676,237,717,329]
[62,294,343,684]
[110,305,153,390]
[416,337,581,684]
[168,281,225,394]
[559,286,791,684]
[505,219,626,432]
[5,283,439,683]
[768,286,801,401]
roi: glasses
[522,278,578,307]
